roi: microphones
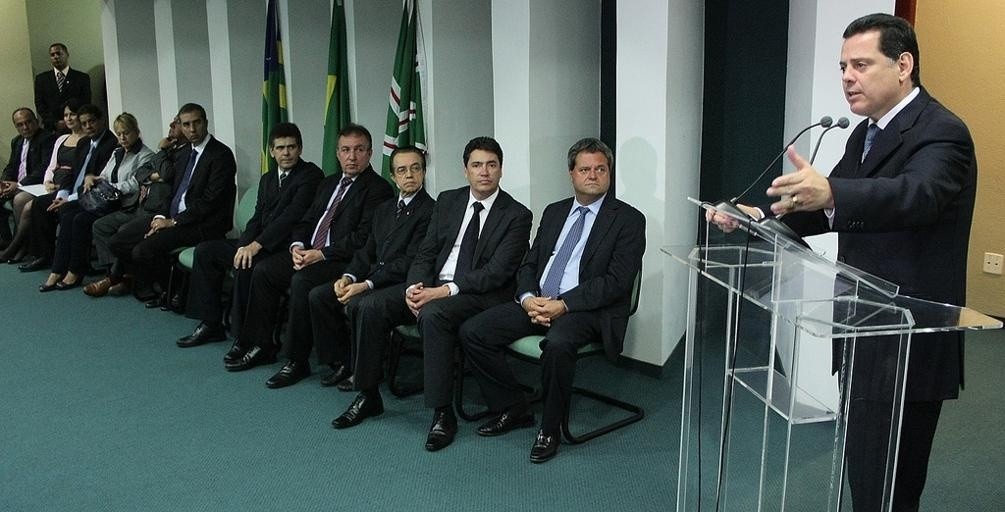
[761,116,850,253]
[713,114,832,220]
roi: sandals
[39,271,83,291]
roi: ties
[395,200,405,223]
[314,178,352,250]
[56,72,65,91]
[170,150,197,218]
[541,207,588,300]
[280,173,286,184]
[19,140,27,179]
[453,202,484,282]
[73,145,95,193]
[861,124,876,163]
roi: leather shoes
[332,395,383,429]
[226,334,277,371]
[145,290,169,310]
[0,251,52,271]
[320,365,354,391]
[266,360,311,388]
[425,409,457,451]
[530,425,560,463]
[83,277,130,297]
[177,322,226,348]
[476,410,535,436]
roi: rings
[155,223,158,226]
[791,192,801,208]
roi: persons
[173,124,326,366]
[146,104,238,315]
[33,41,94,133]
[458,137,647,468]
[706,11,977,511]
[307,145,439,391]
[329,135,533,454]
[39,111,156,292]
[224,124,396,387]
[83,114,192,297]
[0,98,118,272]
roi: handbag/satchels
[77,178,121,213]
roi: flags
[382,0,425,192]
[260,0,288,179]
[322,0,351,179]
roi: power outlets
[982,253,1003,275]
